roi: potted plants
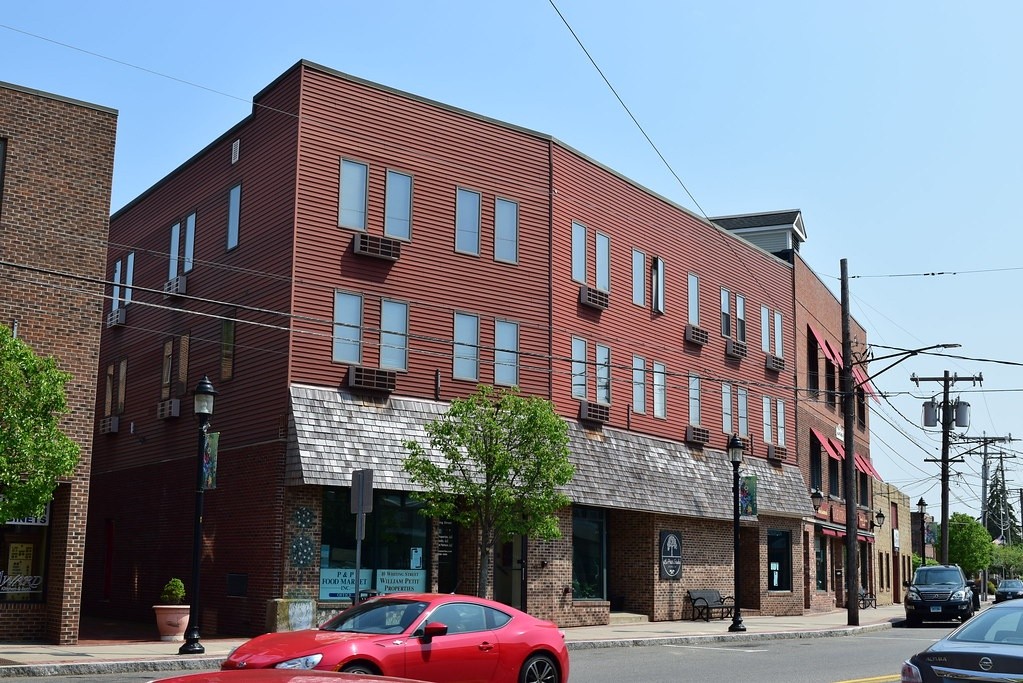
[151,578,191,640]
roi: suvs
[901,563,976,624]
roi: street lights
[915,497,928,566]
[179,373,218,654]
[727,431,749,631]
[844,343,964,629]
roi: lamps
[871,507,885,529]
[825,494,835,501]
[811,486,823,512]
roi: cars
[146,668,437,683]
[222,591,570,683]
[899,598,1023,683]
[994,580,1023,603]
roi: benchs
[687,588,736,623]
[857,587,877,609]
